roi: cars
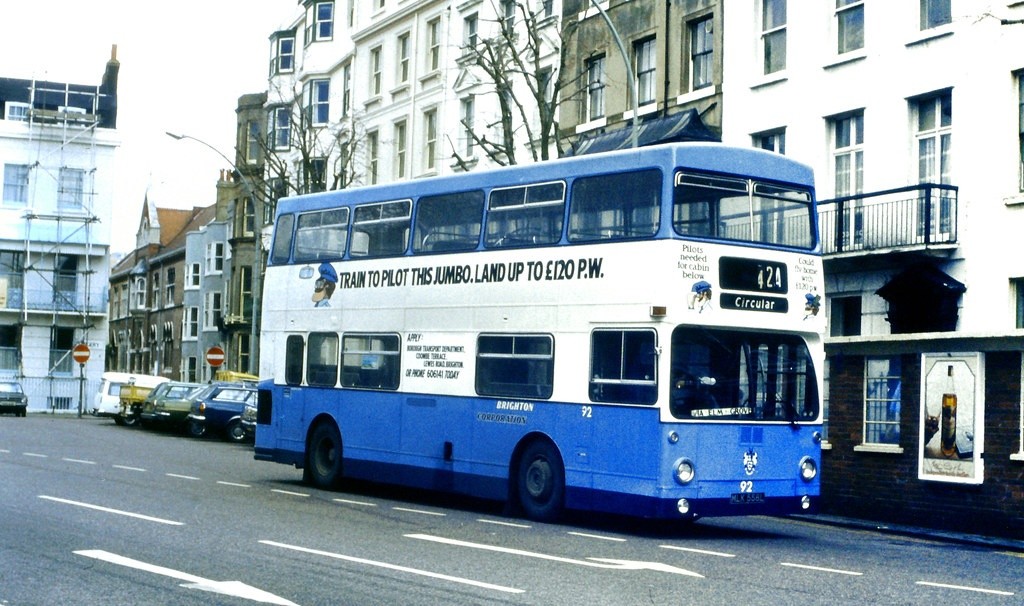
[0,381,27,416]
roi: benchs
[284,225,653,262]
[286,364,551,397]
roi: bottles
[941,365,958,457]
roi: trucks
[119,370,258,426]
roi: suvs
[241,389,256,431]
[139,383,206,431]
[187,385,254,442]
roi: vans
[92,372,187,425]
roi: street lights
[166,131,258,375]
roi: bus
[251,142,828,520]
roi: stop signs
[73,344,90,363]
[206,347,225,366]
